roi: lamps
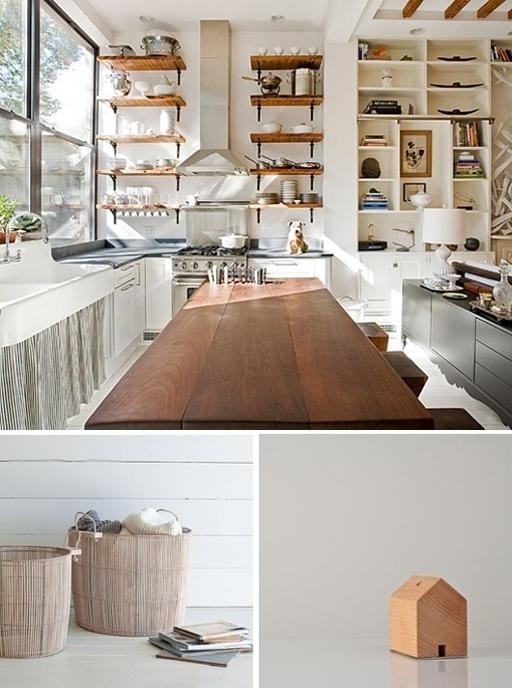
[422,206,470,275]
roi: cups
[131,122,144,134]
[256,267,266,283]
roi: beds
[85,275,435,430]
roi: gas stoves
[179,246,247,256]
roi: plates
[420,283,464,293]
[469,300,511,320]
[301,193,319,204]
[256,192,278,204]
[443,293,467,299]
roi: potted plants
[0,195,18,244]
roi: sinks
[0,284,55,313]
[0,263,112,284]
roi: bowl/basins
[280,179,298,204]
[107,156,127,171]
[161,109,176,135]
[255,47,268,56]
[307,47,319,56]
[290,47,300,56]
[274,47,284,56]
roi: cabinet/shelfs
[144,253,172,332]
[249,55,326,223]
[357,38,492,255]
[249,259,330,292]
[97,55,184,223]
[112,259,145,357]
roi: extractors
[175,20,248,179]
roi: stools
[380,352,427,399]
[426,407,483,431]
[355,322,388,351]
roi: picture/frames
[399,129,432,178]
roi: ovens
[174,257,249,326]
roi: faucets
[0,211,50,265]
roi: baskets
[68,510,192,637]
[0,529,81,659]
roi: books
[454,151,486,178]
[362,96,403,116]
[361,188,393,209]
[453,120,481,147]
[491,43,512,62]
[147,618,253,669]
[359,133,388,147]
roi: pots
[219,233,248,248]
[261,120,282,132]
[259,72,282,90]
[256,159,318,169]
[289,122,316,133]
[139,34,182,57]
